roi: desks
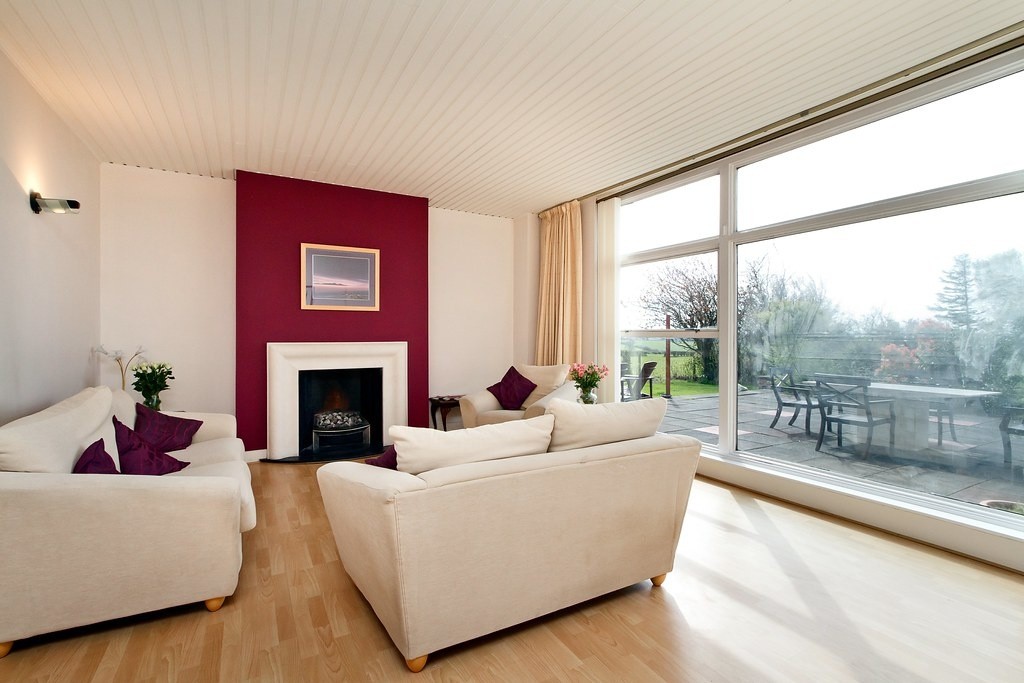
[802,378,1003,454]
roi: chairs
[619,359,658,402]
[997,403,1024,464]
[767,364,833,435]
[813,371,896,460]
[923,362,961,445]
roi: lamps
[29,191,79,218]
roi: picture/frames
[300,242,381,312]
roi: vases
[568,360,609,404]
[127,355,178,412]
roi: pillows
[109,413,192,476]
[72,437,122,473]
[485,366,541,411]
[363,444,399,469]
[133,402,203,452]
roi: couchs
[1,384,257,660]
[456,362,580,426]
[312,393,704,671]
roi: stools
[429,395,465,432]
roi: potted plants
[983,499,1024,518]
